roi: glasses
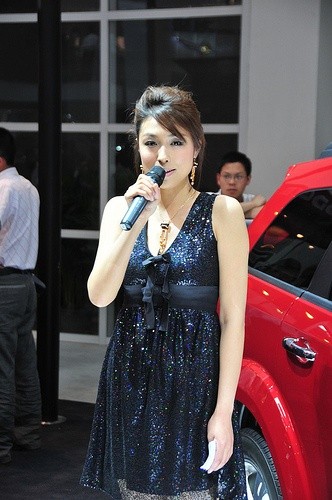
[219,172,248,181]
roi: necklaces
[157,188,198,255]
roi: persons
[0,126,39,461]
[203,150,266,221]
[257,249,314,291]
[86,85,250,500]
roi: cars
[215,155,332,500]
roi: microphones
[120,166,166,231]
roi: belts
[0,266,34,276]
